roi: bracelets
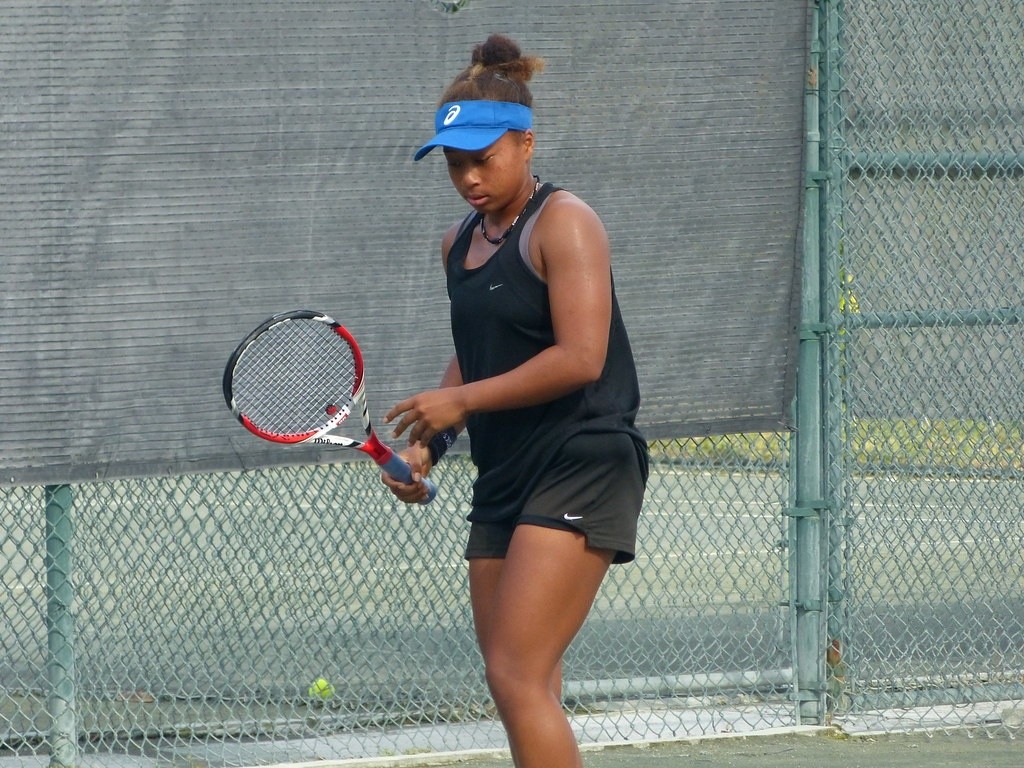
[417,426,458,466]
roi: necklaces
[480,174,540,244]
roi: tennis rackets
[221,309,436,506]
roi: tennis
[308,679,335,701]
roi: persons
[379,33,650,767]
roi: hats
[414,101,534,164]
